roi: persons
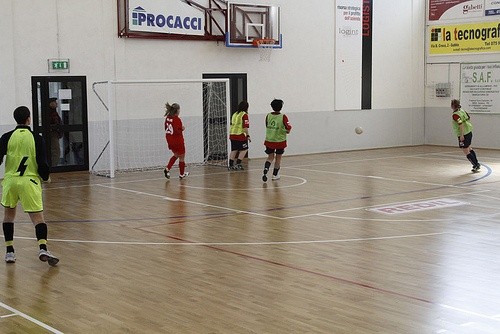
[228,100,253,171]
[49,96,64,166]
[261,98,293,182]
[163,102,191,180]
[1,105,60,266]
[450,98,480,172]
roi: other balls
[355,126,363,135]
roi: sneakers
[272,175,280,179]
[39,249,59,265]
[5,252,16,262]
[228,164,244,170]
[179,171,190,179]
[263,176,267,182]
[471,163,480,171]
[164,168,170,179]
